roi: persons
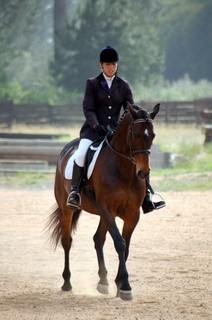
[66,46,166,214]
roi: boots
[137,169,166,214]
[67,159,86,209]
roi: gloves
[94,124,109,135]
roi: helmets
[100,46,119,63]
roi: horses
[44,100,161,301]
